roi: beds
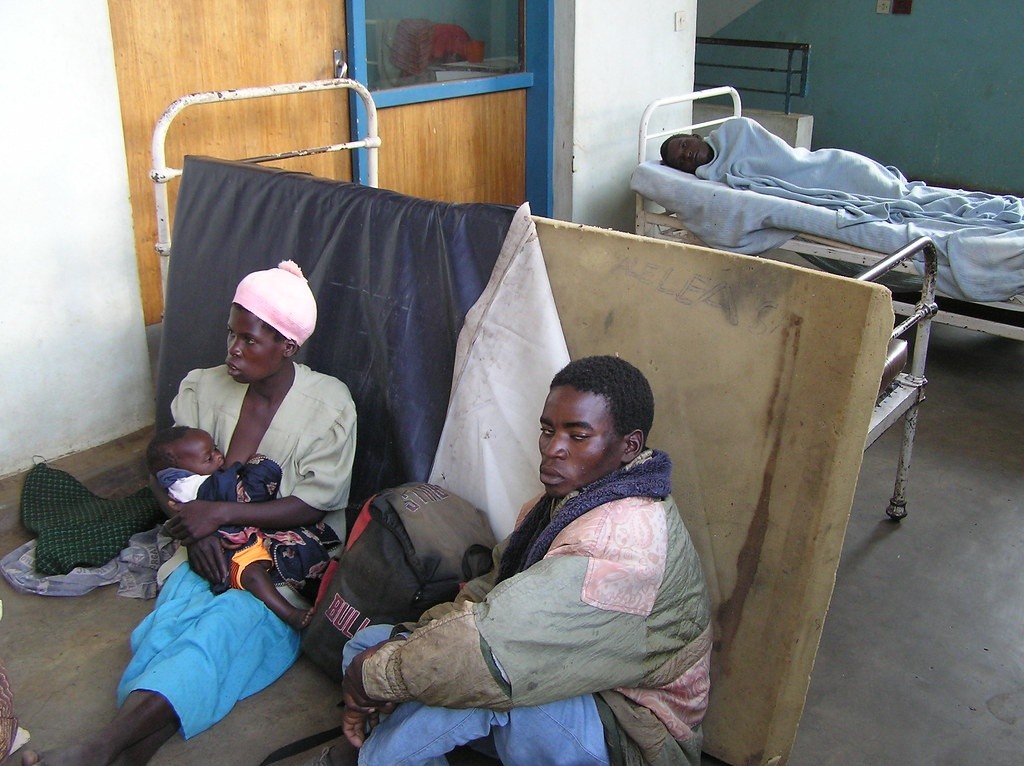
[149,77,940,523]
[635,85,1024,336]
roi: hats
[232,259,317,347]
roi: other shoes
[303,743,336,766]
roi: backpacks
[300,482,497,686]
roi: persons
[146,425,342,636]
[660,133,714,174]
[20,261,357,766]
[343,354,712,766]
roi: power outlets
[877,0,890,13]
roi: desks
[427,56,518,82]
